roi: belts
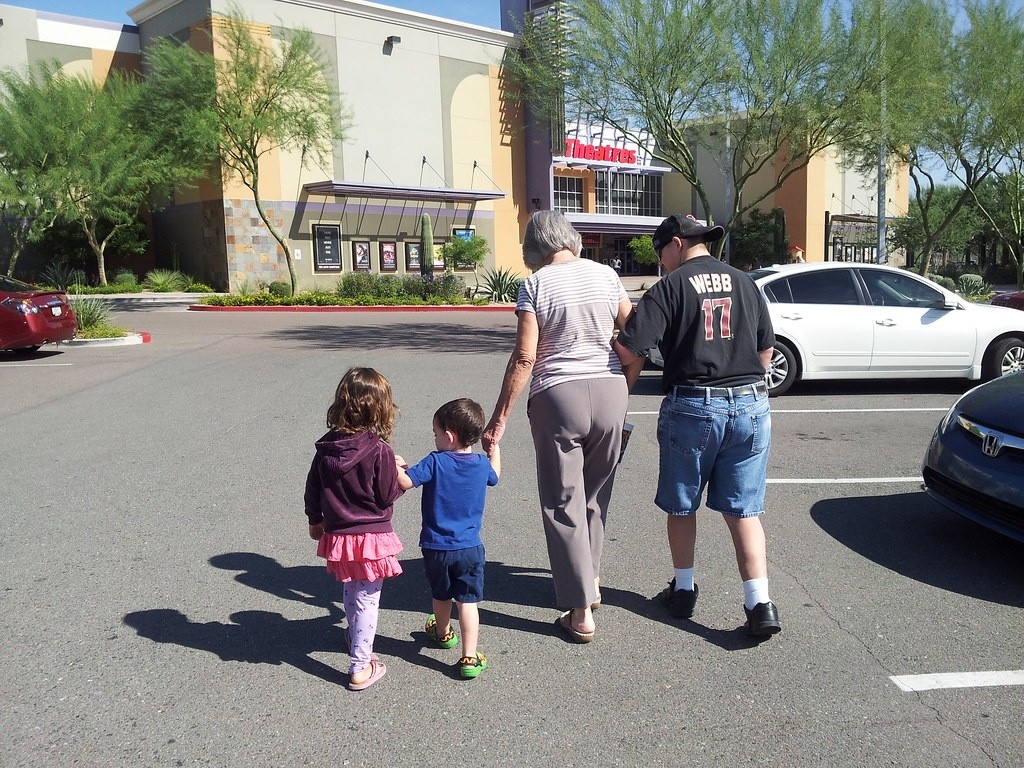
[669,384,767,396]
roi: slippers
[348,660,386,690]
[559,609,594,644]
[592,576,601,608]
[345,626,353,651]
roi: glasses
[656,236,688,260]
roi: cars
[645,259,1024,398]
[1,272,79,355]
[991,288,1024,310]
[921,367,1024,546]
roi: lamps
[387,35,400,44]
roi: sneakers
[743,602,780,635]
[667,576,699,617]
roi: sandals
[459,652,487,678]
[425,614,459,648]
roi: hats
[652,214,725,254]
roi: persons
[611,213,782,638]
[396,398,501,678]
[610,255,622,278]
[304,366,410,692]
[480,210,647,644]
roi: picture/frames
[313,223,477,272]
